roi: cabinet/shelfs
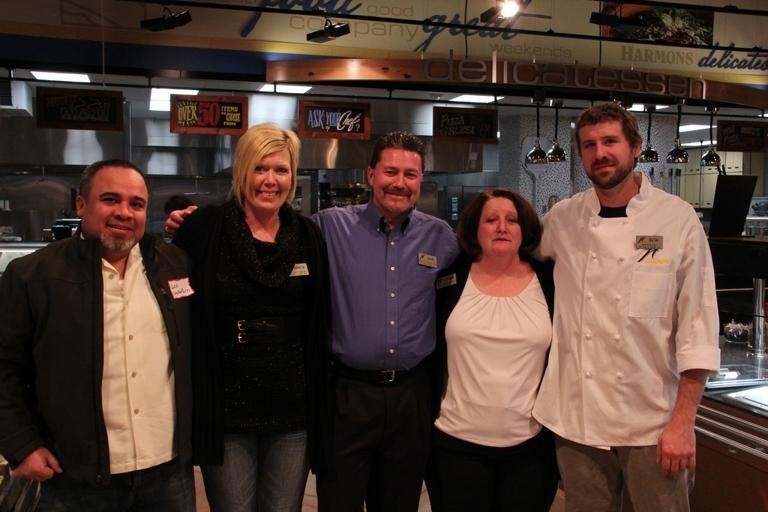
[679,148,764,208]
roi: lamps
[639,103,659,162]
[303,21,353,45]
[700,104,721,167]
[525,86,547,166]
[549,95,567,163]
[666,103,687,164]
[140,9,192,32]
[588,10,655,29]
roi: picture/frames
[296,100,373,139]
[167,95,251,133]
[433,106,500,144]
[35,85,124,131]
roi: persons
[165,131,460,512]
[0,159,196,512]
[175,124,331,512]
[427,189,559,512]
[164,194,194,214]
[536,102,721,512]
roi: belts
[330,362,431,385]
